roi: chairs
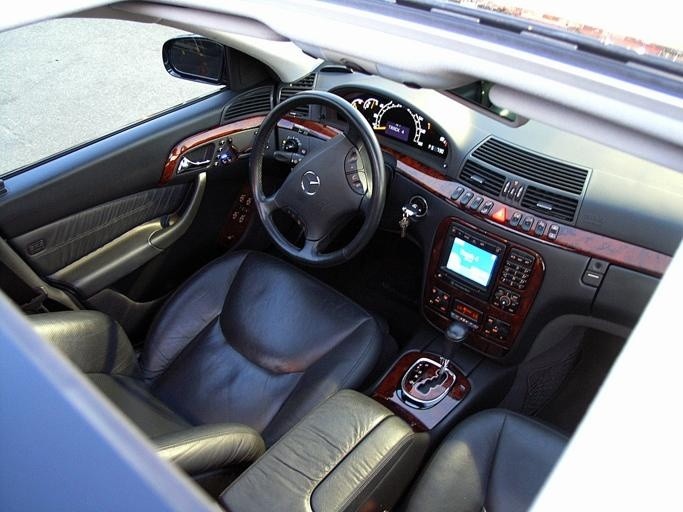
[407,408,570,512]
[28,249,385,505]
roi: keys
[398,216,410,239]
